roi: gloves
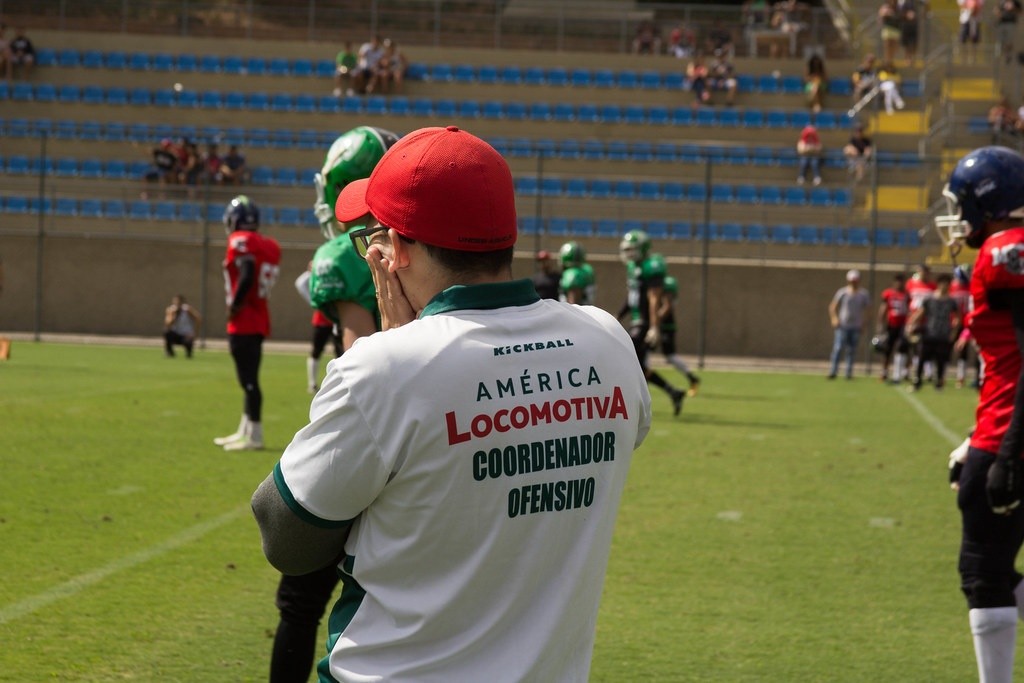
[985,460,1021,519]
[950,437,973,491]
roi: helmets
[222,195,259,234]
[620,230,651,265]
[312,126,400,237]
[934,146,1024,258]
[558,241,584,267]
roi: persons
[333,35,409,96]
[931,145,1024,683]
[988,95,1024,146]
[844,126,874,182]
[876,263,985,389]
[805,54,905,118]
[877,0,1021,64]
[796,125,824,185]
[252,126,653,683]
[740,0,812,57]
[0,22,37,82]
[140,133,247,203]
[827,269,871,379]
[633,20,737,104]
[211,196,280,451]
[616,231,700,417]
[162,295,203,358]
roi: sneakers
[213,431,240,446]
[223,435,265,451]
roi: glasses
[350,226,415,259]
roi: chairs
[0,46,991,251]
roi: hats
[848,270,860,282]
[334,126,517,251]
[536,250,549,260]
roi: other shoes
[828,372,982,391]
[687,378,700,396]
[674,390,685,416]
